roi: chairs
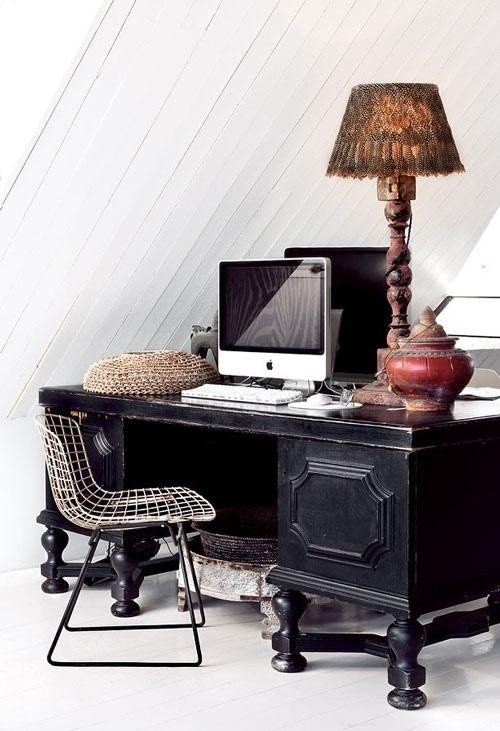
[33,414,217,668]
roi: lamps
[323,82,468,410]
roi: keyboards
[181,383,302,405]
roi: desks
[35,385,500,711]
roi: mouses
[307,394,333,406]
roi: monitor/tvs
[284,246,392,388]
[217,257,333,399]
